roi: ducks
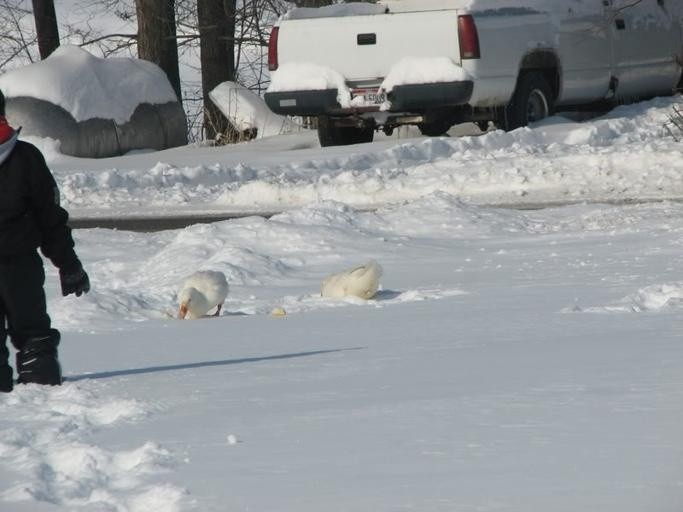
[319,258,383,300]
[175,269,230,319]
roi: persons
[1,90,90,393]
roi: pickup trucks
[264,0,683,147]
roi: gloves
[50,247,90,297]
